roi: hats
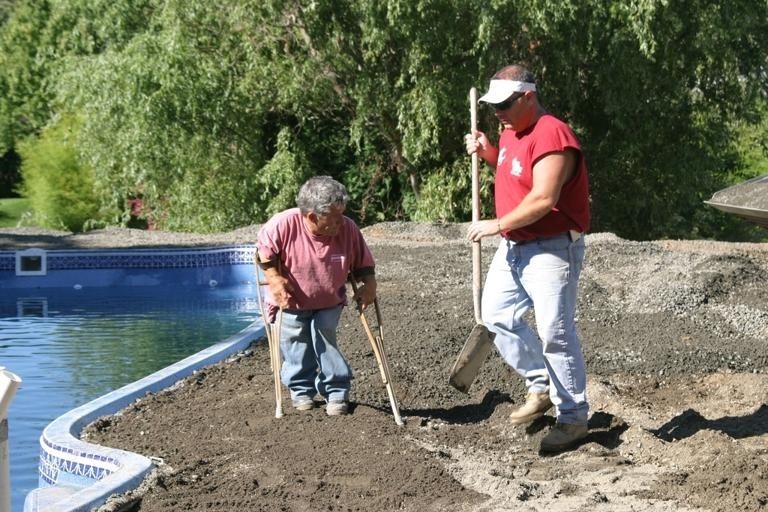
[477,79,537,104]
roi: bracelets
[497,218,501,232]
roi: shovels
[448,87,497,394]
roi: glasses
[487,93,524,110]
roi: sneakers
[541,421,588,452]
[326,402,348,416]
[509,391,553,424]
[293,399,314,411]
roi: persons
[254,174,379,414]
[460,64,594,452]
